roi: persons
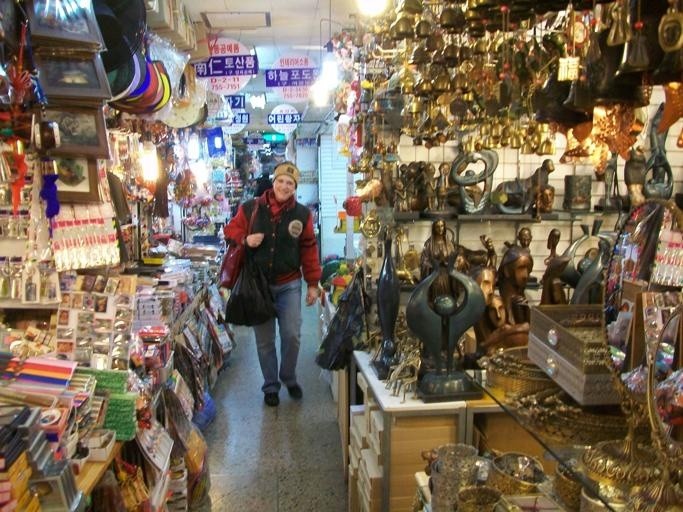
[220,160,320,405]
[419,218,572,357]
[393,159,556,213]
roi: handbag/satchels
[217,196,260,288]
[224,263,278,327]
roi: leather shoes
[265,392,279,406]
[287,384,305,400]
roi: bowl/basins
[430,443,544,510]
[555,462,626,512]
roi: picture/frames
[28,0,113,206]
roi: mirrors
[581,198,683,512]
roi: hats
[272,161,301,191]
